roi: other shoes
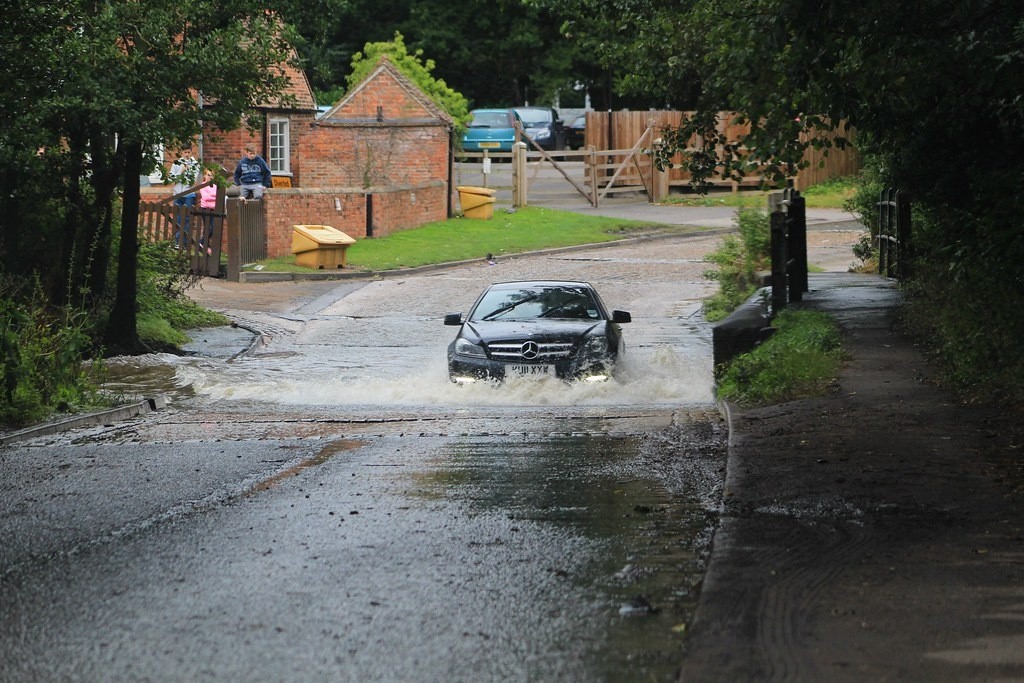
[169,243,181,249]
[199,241,212,256]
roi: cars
[516,107,565,161]
[444,280,631,387]
[461,109,524,161]
[563,112,585,150]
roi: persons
[170,144,218,256]
[234,145,271,199]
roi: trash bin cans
[456,186,496,220]
[291,223,357,269]
